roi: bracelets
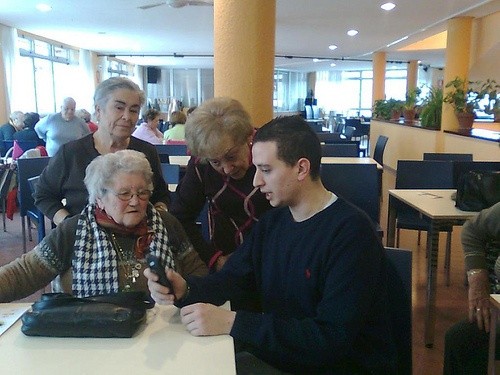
[467,268,487,275]
[63,213,74,219]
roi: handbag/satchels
[20,290,155,338]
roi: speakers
[147,67,157,83]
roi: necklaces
[111,230,134,267]
[109,233,137,287]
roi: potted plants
[444,78,492,129]
[419,89,440,130]
[402,92,420,123]
[388,100,403,121]
[489,97,500,122]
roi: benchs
[152,121,383,237]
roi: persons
[144,114,408,375]
[443,204,500,375]
[0,76,273,311]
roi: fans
[139,0,214,11]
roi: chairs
[0,117,207,253]
[334,109,500,295]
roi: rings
[476,308,481,311]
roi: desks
[169,155,191,166]
[0,298,238,375]
[487,293,500,374]
[321,158,382,169]
[387,183,483,345]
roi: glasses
[118,190,154,201]
